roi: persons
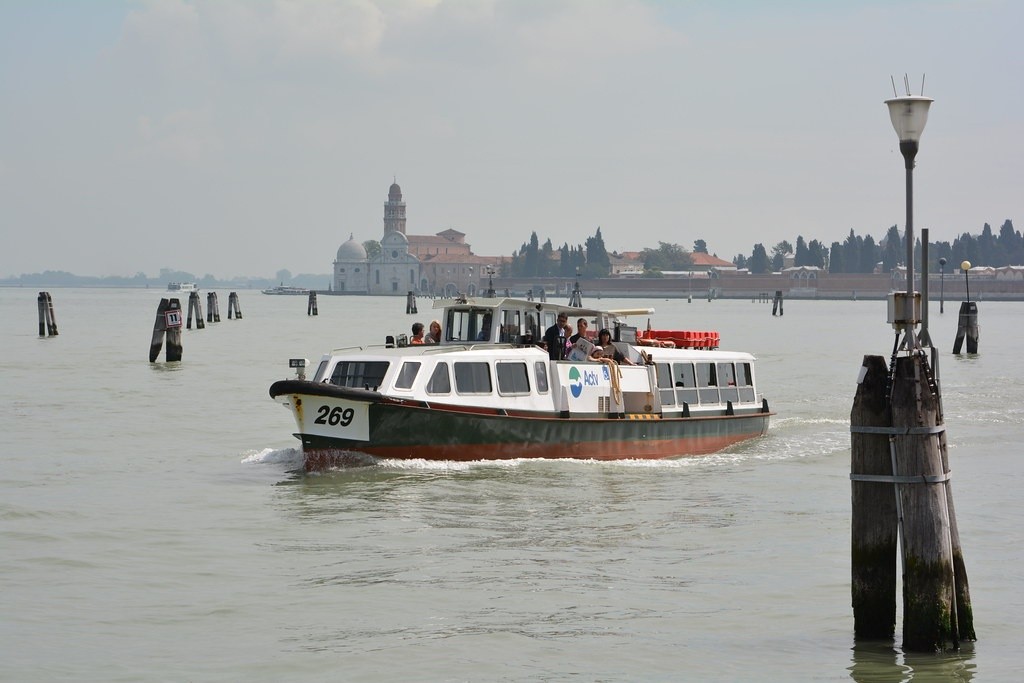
[411,323,426,344]
[587,346,612,363]
[424,320,441,344]
[476,322,492,341]
[595,329,634,365]
[546,312,594,361]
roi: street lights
[940,258,946,313]
[883,74,932,359]
[960,260,972,303]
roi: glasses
[557,319,565,323]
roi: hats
[590,345,604,355]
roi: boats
[261,282,311,295]
[269,300,776,471]
[166,281,200,293]
